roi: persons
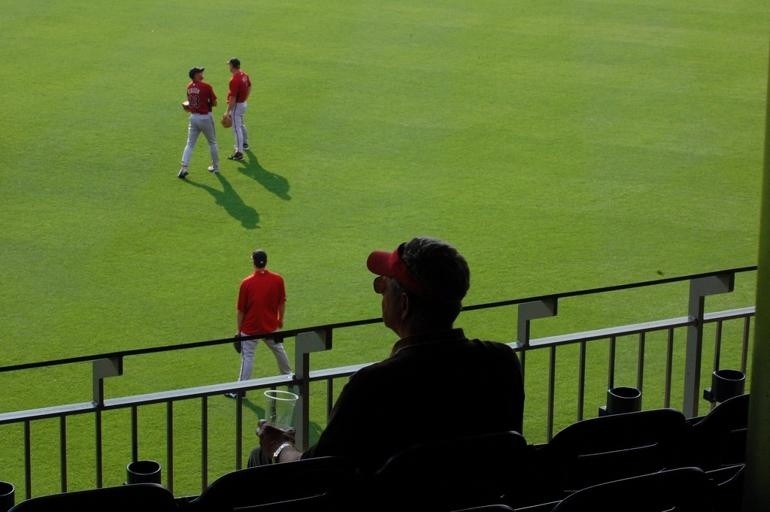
[224,58,252,162]
[224,250,294,400]
[245,234,526,469]
[176,65,221,181]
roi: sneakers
[208,166,219,174]
[178,169,188,178]
[224,393,246,400]
[189,68,204,77]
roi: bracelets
[272,438,296,464]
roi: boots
[243,144,249,150]
[228,151,244,160]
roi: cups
[263,390,299,433]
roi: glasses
[374,276,387,293]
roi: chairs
[0,389,749,511]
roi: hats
[367,238,471,301]
[253,251,267,259]
[226,57,240,65]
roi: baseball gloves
[221,113,232,128]
[234,334,241,354]
[182,101,190,111]
